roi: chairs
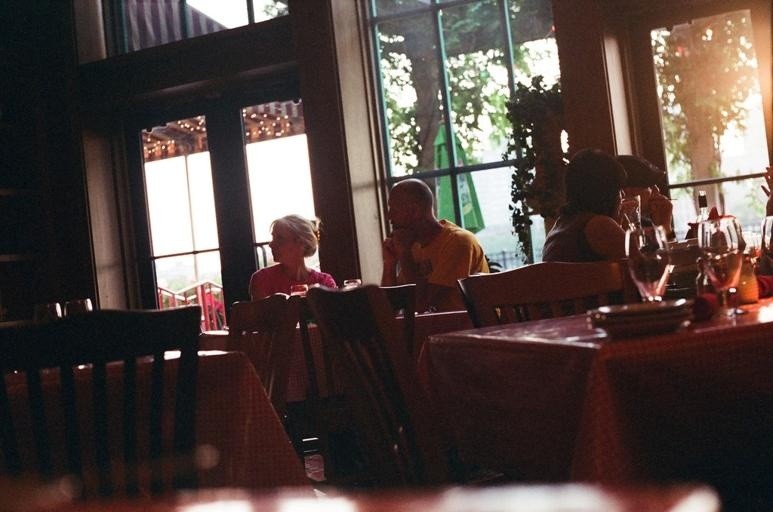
[3,320,100,500]
[226,293,315,405]
[456,258,633,327]
[46,306,203,502]
[299,278,419,488]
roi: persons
[614,153,679,256]
[542,146,640,317]
[759,163,773,257]
[379,176,501,317]
[247,213,337,302]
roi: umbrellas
[432,119,485,234]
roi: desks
[197,309,475,488]
[1,349,318,488]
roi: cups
[64,298,93,318]
[289,284,309,297]
[34,301,62,322]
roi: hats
[616,155,665,188]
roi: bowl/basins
[589,297,694,337]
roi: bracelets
[666,228,676,241]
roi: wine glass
[696,217,745,317]
[622,195,643,230]
[625,225,672,300]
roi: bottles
[696,191,710,220]
